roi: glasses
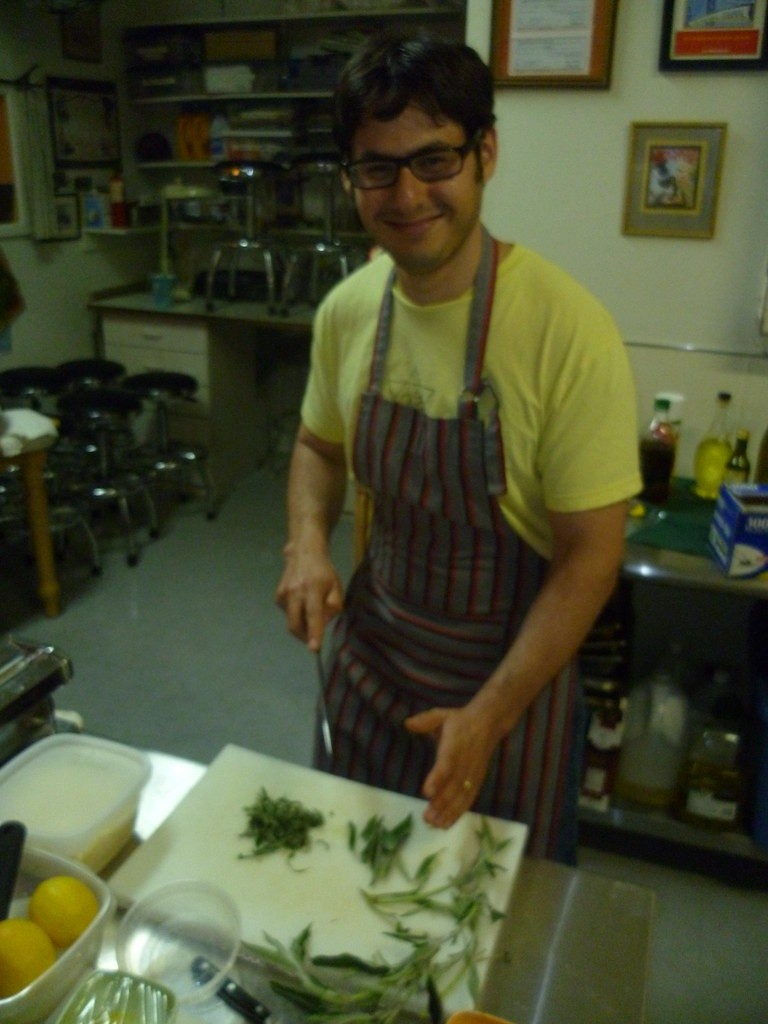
[336,124,484,191]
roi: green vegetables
[238,785,515,1024]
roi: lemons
[0,873,100,1001]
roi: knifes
[314,650,335,760]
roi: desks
[87,289,319,492]
[0,418,60,619]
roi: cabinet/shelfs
[576,474,768,891]
[85,7,457,240]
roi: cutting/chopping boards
[102,742,530,1019]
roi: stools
[162,183,215,273]
[206,151,350,316]
[1,359,218,577]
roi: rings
[462,780,474,791]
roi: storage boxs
[704,482,768,583]
[203,27,277,93]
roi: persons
[276,28,642,867]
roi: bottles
[720,429,751,486]
[689,392,734,500]
[615,668,691,806]
[633,399,678,509]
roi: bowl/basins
[116,877,244,1015]
[0,843,112,1024]
[1,731,154,875]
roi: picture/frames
[61,0,101,62]
[660,0,768,72]
[46,194,82,242]
[622,120,728,240]
